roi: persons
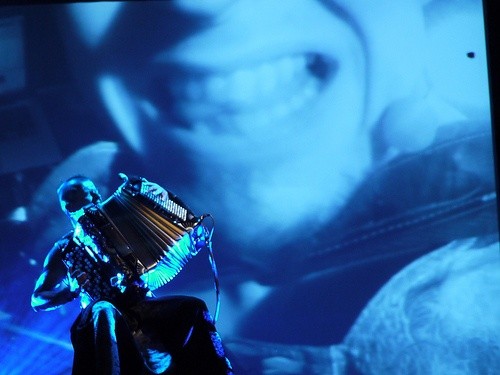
[31,174,233,374]
[3,0,499,375]
